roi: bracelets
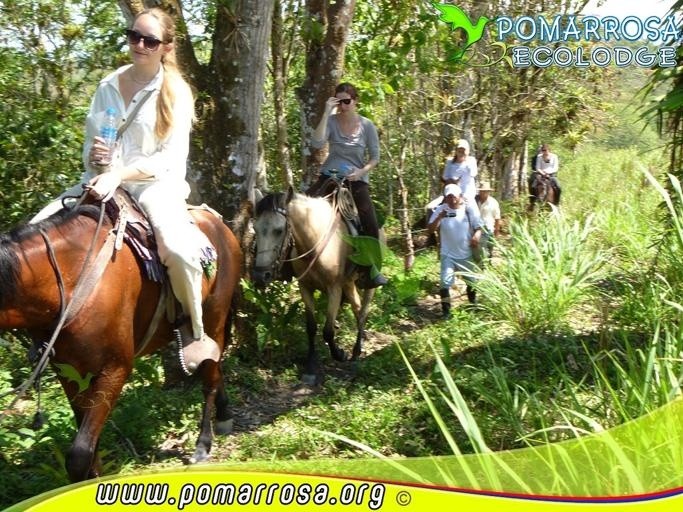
[494,227,499,230]
[324,111,329,114]
[437,217,441,221]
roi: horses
[0,202,244,483]
[247,186,387,388]
[528,174,556,213]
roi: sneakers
[170,335,215,368]
[359,270,387,289]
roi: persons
[528,147,542,185]
[440,138,481,213]
[470,180,500,268]
[276,82,386,286]
[527,144,562,211]
[28,6,223,377]
[426,183,481,318]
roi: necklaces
[129,68,151,85]
[340,124,354,136]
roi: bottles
[340,163,353,175]
[92,108,117,168]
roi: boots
[467,287,478,311]
[439,289,452,320]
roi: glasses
[335,98,353,105]
[125,29,166,51]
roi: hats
[476,181,495,192]
[455,139,470,150]
[443,183,462,198]
